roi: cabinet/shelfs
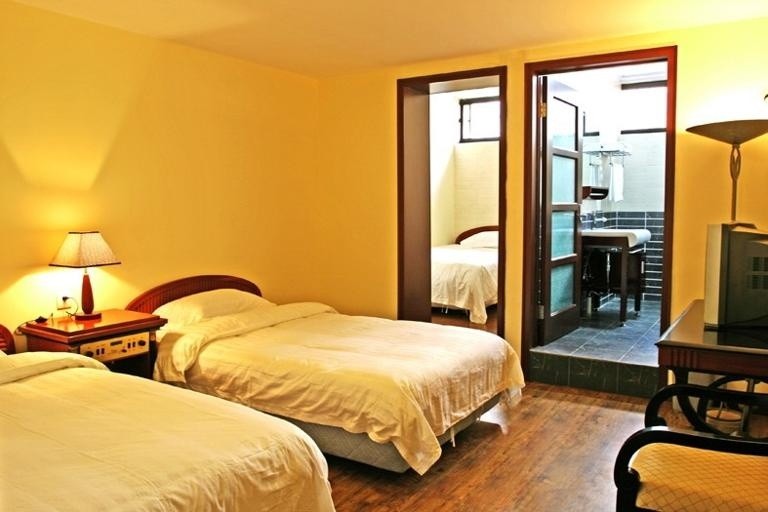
[579,237,646,326]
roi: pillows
[460,231,499,248]
[154,286,276,323]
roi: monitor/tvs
[703,222,768,328]
[704,328,768,350]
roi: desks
[655,299,768,439]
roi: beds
[431,225,500,324]
[1,323,341,512]
[124,274,525,474]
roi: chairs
[614,382,768,512]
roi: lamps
[680,119,768,430]
[48,232,121,320]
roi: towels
[608,164,624,203]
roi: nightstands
[19,308,167,380]
